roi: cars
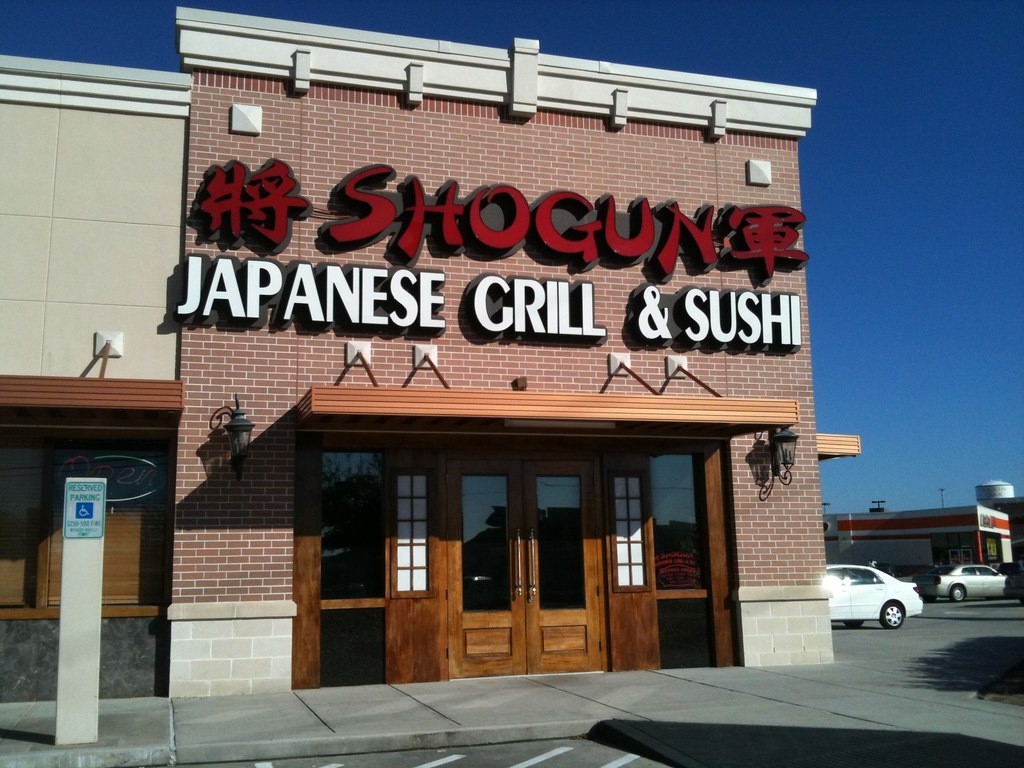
[827,563,924,629]
[911,564,1007,601]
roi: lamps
[771,427,799,485]
[223,392,255,481]
